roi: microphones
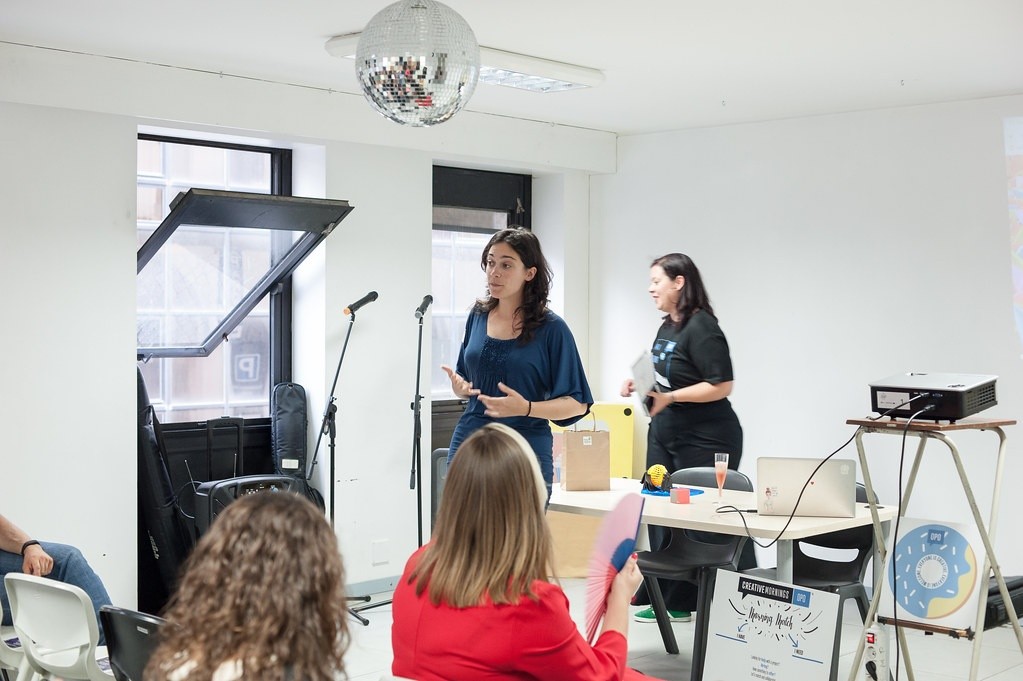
[414,295,433,319]
[343,291,378,315]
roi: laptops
[756,457,856,516]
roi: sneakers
[634,604,692,622]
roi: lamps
[356,1,479,127]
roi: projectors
[870,371,999,422]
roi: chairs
[98,604,172,681]
[628,465,754,680]
[734,478,881,681]
[4,571,115,681]
[0,598,44,681]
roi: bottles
[865,612,889,681]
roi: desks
[543,475,898,681]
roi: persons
[140,488,351,681]
[620,253,759,623]
[0,516,115,647]
[442,227,593,519]
[392,422,668,681]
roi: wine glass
[713,453,729,504]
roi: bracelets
[526,401,532,417]
[21,540,40,555]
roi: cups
[670,488,690,504]
